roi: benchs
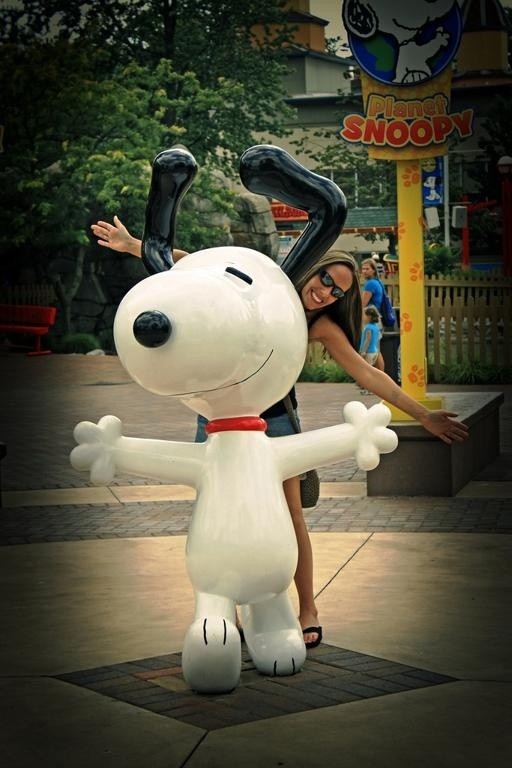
[1,303,58,357]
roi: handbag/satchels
[300,470,320,509]
[380,295,396,327]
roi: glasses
[317,267,346,300]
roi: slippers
[302,626,324,651]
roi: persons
[87,214,473,650]
[355,305,382,397]
[359,256,386,372]
